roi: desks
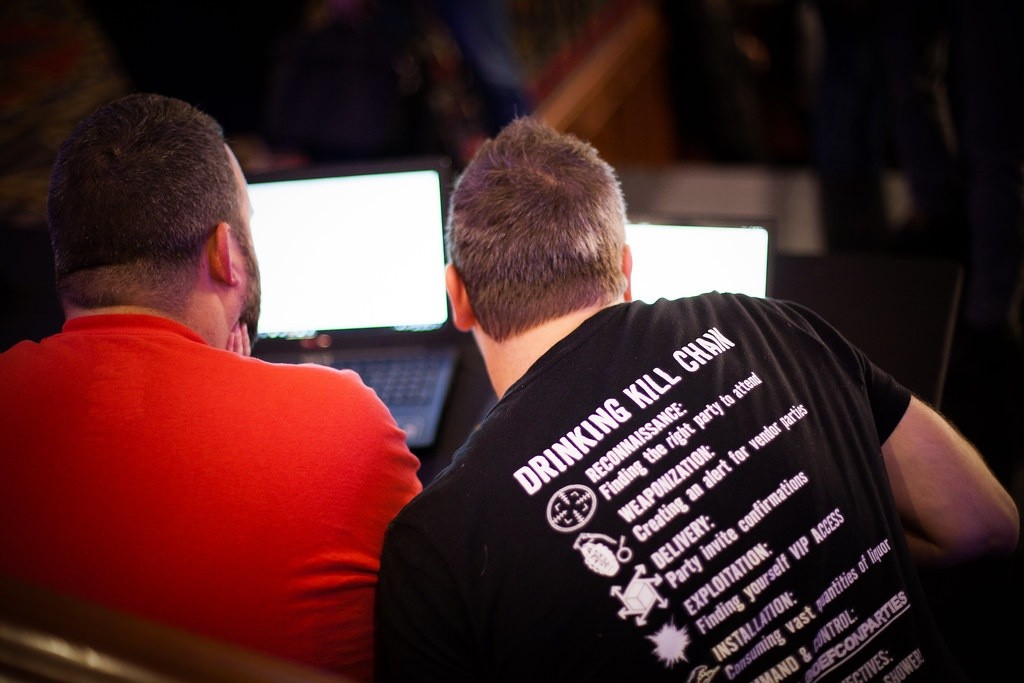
[248,248,972,496]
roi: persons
[373,116,1020,683]
[0,95,423,683]
[0,0,825,233]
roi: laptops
[245,157,463,456]
[627,210,777,306]
